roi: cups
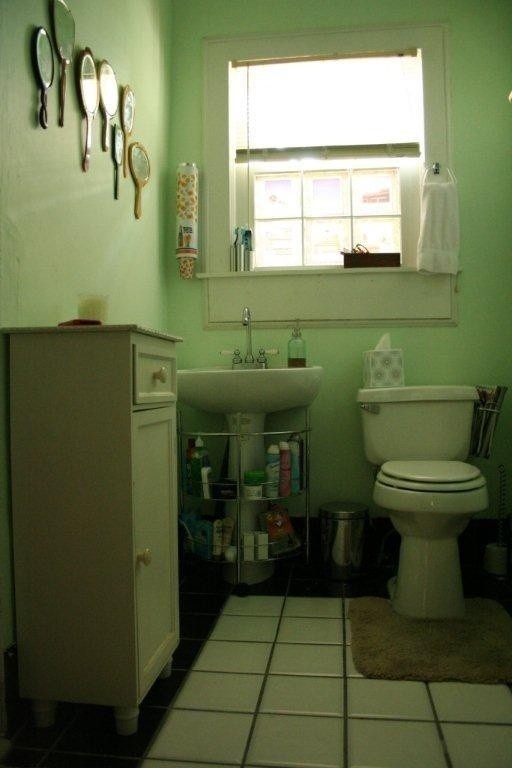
[230,246,255,274]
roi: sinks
[177,365,325,416]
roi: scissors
[351,244,369,254]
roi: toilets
[356,385,492,622]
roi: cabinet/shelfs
[2,328,182,738]
[176,424,318,586]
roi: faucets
[242,306,256,364]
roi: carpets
[346,591,512,684]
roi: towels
[416,183,462,276]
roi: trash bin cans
[318,502,370,582]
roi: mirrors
[32,43,153,221]
